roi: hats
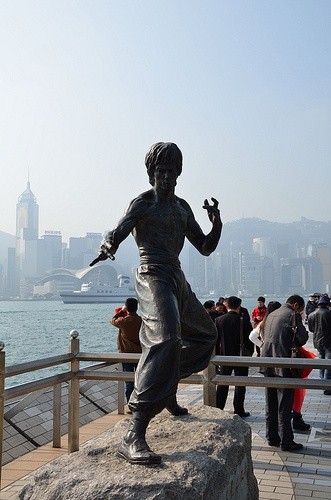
[268,301,281,313]
[317,295,330,305]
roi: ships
[59,274,138,305]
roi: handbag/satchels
[239,345,251,356]
[290,347,304,378]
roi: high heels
[292,414,310,431]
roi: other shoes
[280,440,303,451]
[269,439,283,446]
[235,411,250,418]
[323,390,331,395]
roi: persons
[202,289,331,394]
[247,301,310,431]
[213,295,250,418]
[108,297,143,414]
[257,295,309,452]
[87,140,223,466]
